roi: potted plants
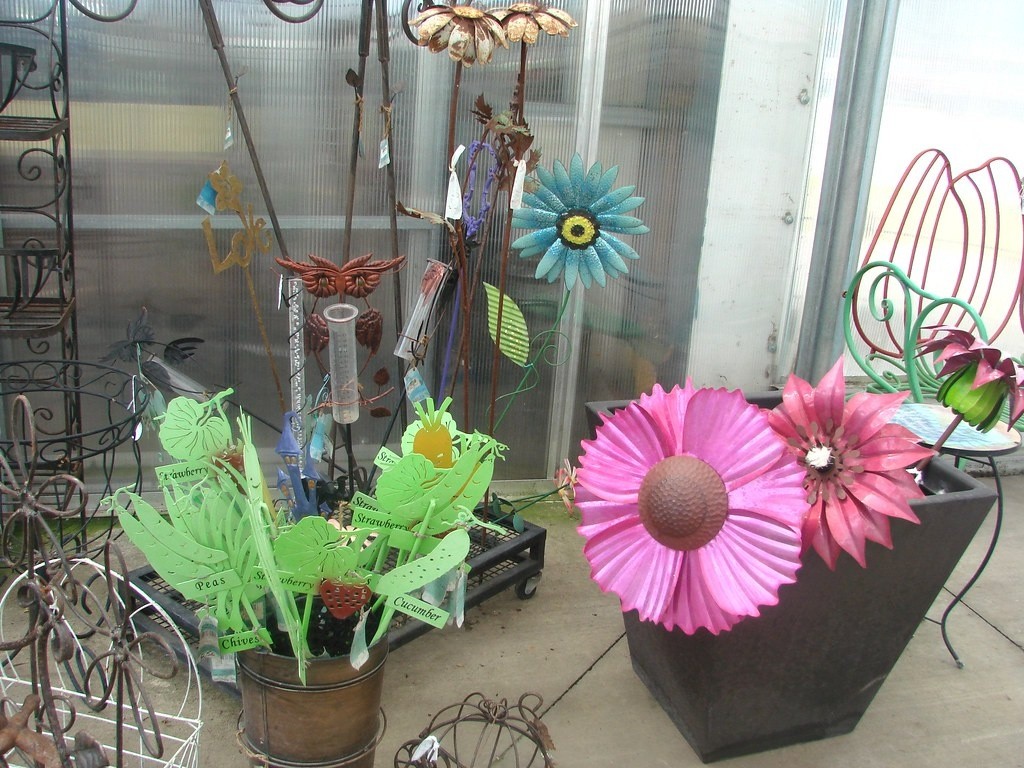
[578,380,996,759]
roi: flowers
[568,359,929,638]
